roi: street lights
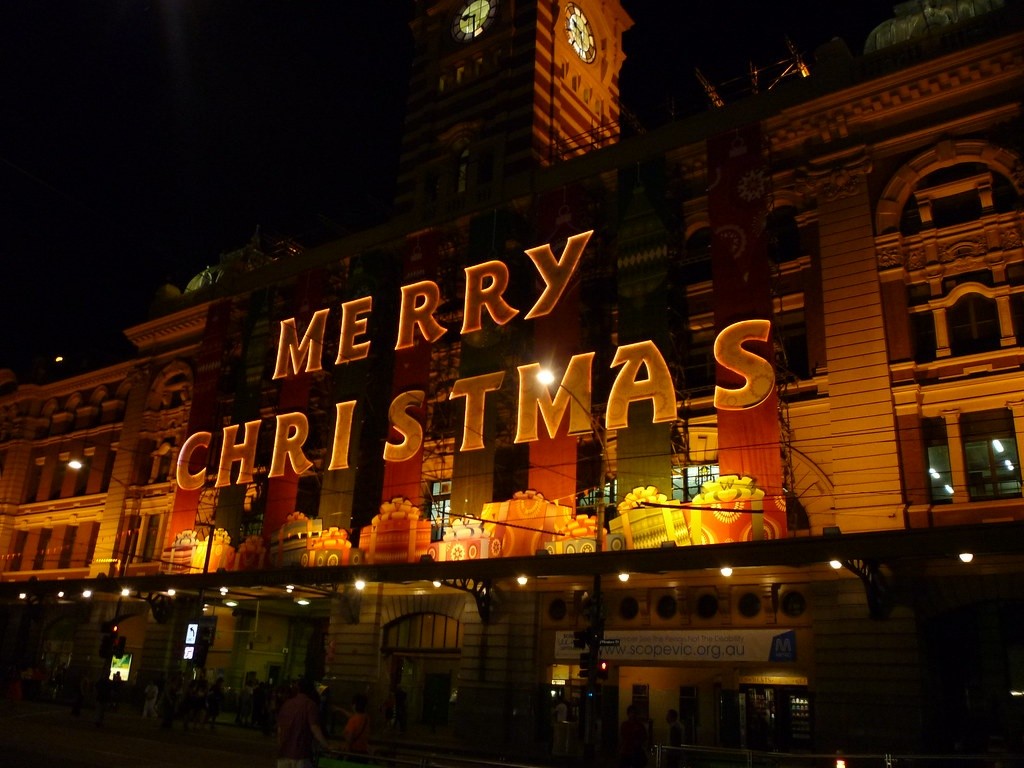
[183,522,216,732]
[68,461,143,727]
[538,368,607,768]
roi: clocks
[563,2,599,64]
[451,1,496,42]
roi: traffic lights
[574,630,586,649]
[580,652,592,678]
[194,641,209,668]
[113,636,125,658]
[598,659,608,679]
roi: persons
[616,705,648,768]
[275,679,369,768]
[666,710,683,768]
[94,672,120,726]
[47,663,73,706]
[231,671,273,730]
[138,665,224,741]
[721,705,791,751]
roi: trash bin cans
[551,719,583,761]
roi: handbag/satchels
[633,743,649,768]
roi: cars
[44,669,78,705]
